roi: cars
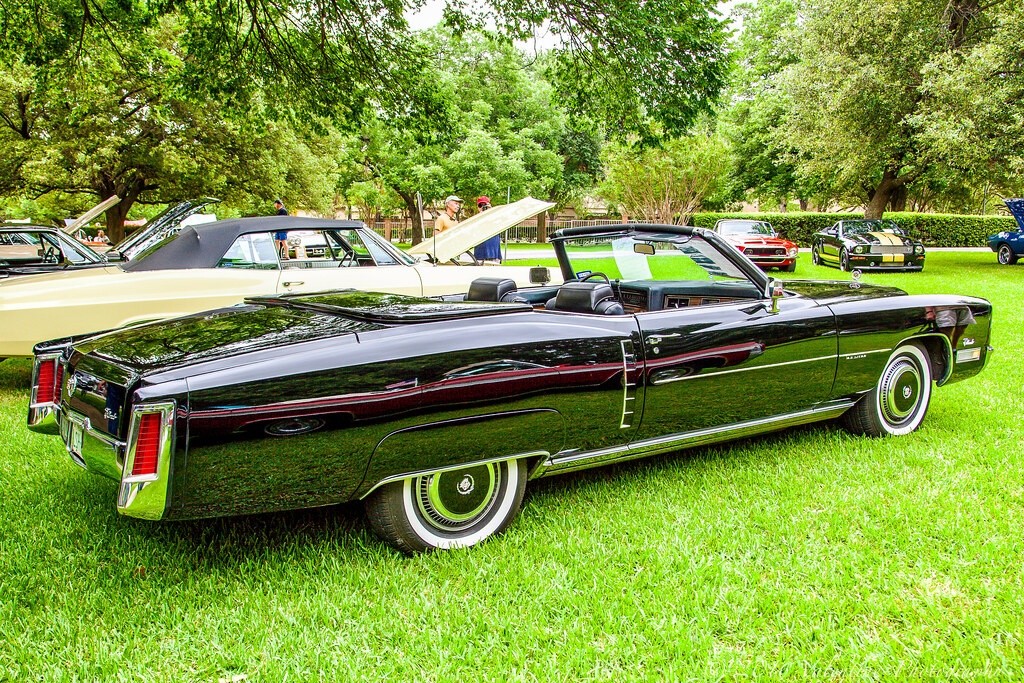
[7,193,122,234]
[0,192,559,368]
[712,218,800,273]
[26,221,993,555]
[985,197,1024,266]
[0,193,221,283]
[810,218,926,275]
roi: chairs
[545,281,624,316]
[462,277,529,303]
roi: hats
[445,195,464,204]
[477,196,490,204]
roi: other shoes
[281,256,290,260]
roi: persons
[474,196,502,264]
[94,228,110,242]
[433,196,464,260]
[274,200,290,260]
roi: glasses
[478,203,486,208]
